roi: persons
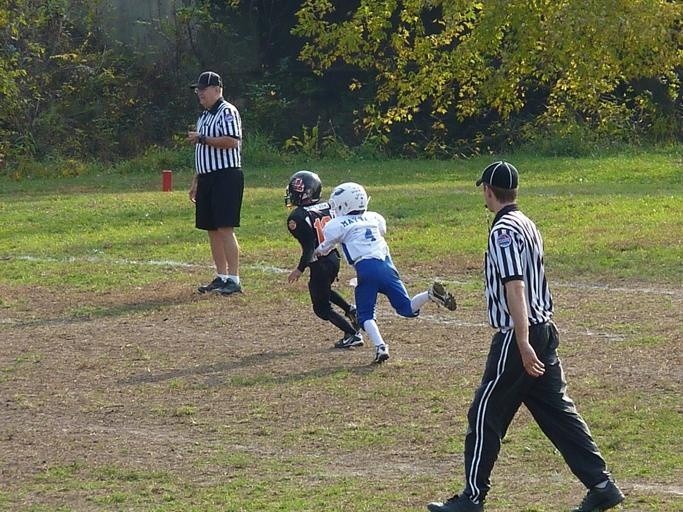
[284,169,368,352]
[309,181,461,364]
[184,70,247,296]
[426,161,627,512]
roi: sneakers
[335,304,364,348]
[374,344,389,363]
[427,494,483,512]
[571,480,625,512]
[428,282,456,310]
[198,277,240,294]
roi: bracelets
[199,133,206,145]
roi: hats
[190,72,223,88]
[476,161,518,189]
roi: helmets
[285,169,321,206]
[328,182,368,218]
